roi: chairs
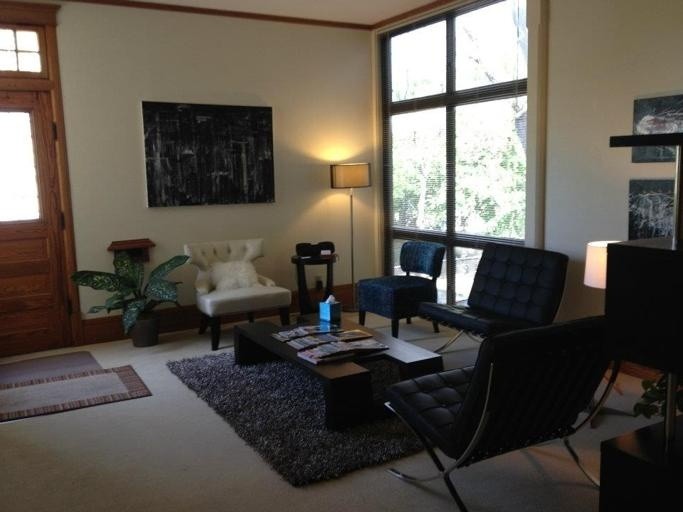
[182,236,293,350]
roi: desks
[292,255,339,314]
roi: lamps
[581,239,621,290]
[330,162,371,315]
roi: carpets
[162,350,435,490]
[1,350,153,424]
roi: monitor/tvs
[141,100,275,207]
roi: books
[270,321,391,366]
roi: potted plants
[70,250,189,348]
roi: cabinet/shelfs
[597,135,683,512]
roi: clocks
[207,258,258,293]
